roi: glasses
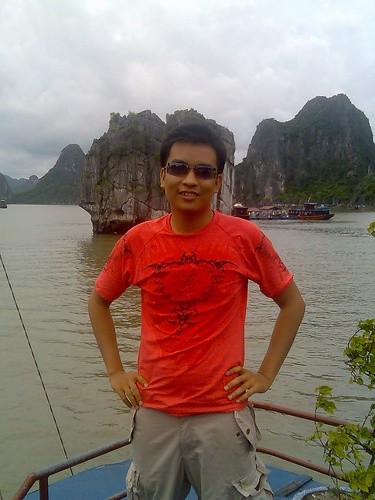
[164,163,219,180]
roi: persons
[256,211,274,218]
[89,125,305,500]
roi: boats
[0,200,7,208]
[232,203,335,220]
